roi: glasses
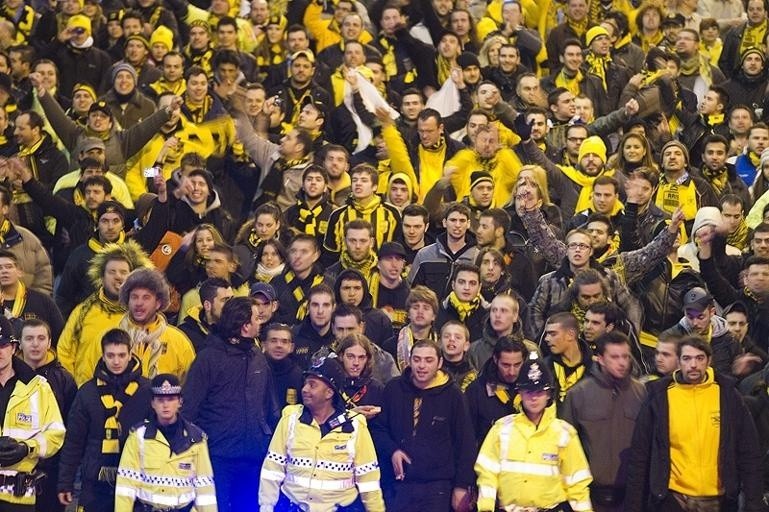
[565,243,592,250]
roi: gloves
[392,22,410,42]
[513,113,535,140]
[0,436,26,469]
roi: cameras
[274,96,284,107]
[72,27,86,34]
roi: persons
[646,336,682,382]
[477,249,530,336]
[270,99,292,144]
[385,172,413,215]
[521,177,685,287]
[546,0,600,75]
[460,108,488,144]
[696,226,769,350]
[396,205,435,278]
[32,0,84,51]
[261,24,331,92]
[43,135,136,236]
[481,36,506,65]
[674,28,726,110]
[0,317,79,512]
[0,20,19,56]
[100,10,125,50]
[258,355,387,512]
[334,268,393,347]
[178,66,235,159]
[83,0,111,49]
[624,336,765,511]
[538,39,612,120]
[547,87,614,152]
[543,312,594,403]
[716,194,753,252]
[11,58,71,143]
[181,19,216,86]
[652,57,734,167]
[348,125,382,170]
[763,204,769,224]
[280,165,340,244]
[0,111,69,250]
[248,0,269,42]
[726,121,769,187]
[385,282,442,373]
[477,81,508,126]
[588,0,630,22]
[624,115,647,137]
[253,91,327,156]
[467,292,542,368]
[747,146,769,204]
[677,206,741,272]
[254,14,289,80]
[56,328,152,511]
[326,219,376,285]
[0,185,53,296]
[656,13,685,52]
[667,0,703,31]
[169,0,259,54]
[623,171,704,337]
[317,12,382,70]
[367,5,401,79]
[366,243,411,327]
[303,0,373,54]
[721,45,769,125]
[133,0,179,42]
[336,335,383,422]
[579,301,621,352]
[449,10,483,55]
[661,287,744,382]
[374,107,418,203]
[29,71,184,180]
[248,282,278,348]
[423,165,508,235]
[665,52,697,112]
[508,73,550,111]
[582,26,632,112]
[652,139,721,219]
[713,222,769,289]
[548,124,588,202]
[56,176,169,322]
[718,0,769,80]
[607,12,644,76]
[561,332,648,512]
[213,16,257,83]
[304,0,370,40]
[148,51,187,102]
[169,168,232,246]
[581,22,626,66]
[504,163,562,228]
[267,48,332,132]
[510,107,559,167]
[57,240,146,389]
[54,173,138,262]
[125,191,158,236]
[696,0,747,39]
[329,36,417,108]
[483,0,541,73]
[409,107,465,205]
[488,46,530,101]
[179,297,281,512]
[508,183,564,276]
[316,306,399,388]
[234,201,293,282]
[699,134,752,215]
[367,339,477,512]
[443,127,524,208]
[721,298,763,362]
[8,157,77,220]
[323,145,352,206]
[327,64,402,152]
[108,10,148,62]
[74,267,198,387]
[340,65,474,158]
[698,19,723,65]
[409,0,453,48]
[0,53,27,104]
[475,207,538,302]
[99,62,154,131]
[727,104,754,158]
[220,79,312,220]
[178,277,234,354]
[468,338,534,452]
[260,320,307,408]
[438,262,491,327]
[365,60,401,112]
[55,157,105,206]
[8,44,36,94]
[474,355,595,512]
[270,234,337,326]
[39,12,113,98]
[512,111,627,220]
[244,83,265,127]
[619,46,669,145]
[0,72,22,121]
[238,238,288,296]
[172,225,243,298]
[97,31,161,102]
[113,372,219,512]
[630,4,663,53]
[291,284,334,369]
[405,202,480,301]
[146,25,174,67]
[617,132,658,176]
[0,106,19,157]
[146,136,205,194]
[0,0,41,47]
[177,246,237,327]
[762,31,769,59]
[206,50,248,114]
[437,320,479,395]
[546,269,648,378]
[482,89,640,149]
[57,80,122,164]
[392,15,462,98]
[1,251,66,349]
[123,91,214,202]
[564,176,624,234]
[321,163,402,267]
[455,52,483,113]
[527,228,644,337]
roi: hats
[70,79,99,102]
[249,281,278,304]
[78,135,107,153]
[379,244,407,264]
[467,170,495,192]
[737,45,767,67]
[267,14,289,32]
[289,49,317,67]
[722,299,748,320]
[758,147,769,167]
[151,23,174,52]
[513,357,559,394]
[68,16,92,35]
[586,25,611,48]
[123,32,151,52]
[576,135,609,165]
[87,100,112,119]
[0,318,19,349]
[111,62,140,86]
[147,373,184,397]
[658,137,690,166]
[455,49,480,70]
[105,9,126,25]
[683,287,715,312]
[302,354,348,396]
[301,93,330,125]
[186,19,212,37]
[189,168,216,193]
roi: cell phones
[144,167,158,177]
